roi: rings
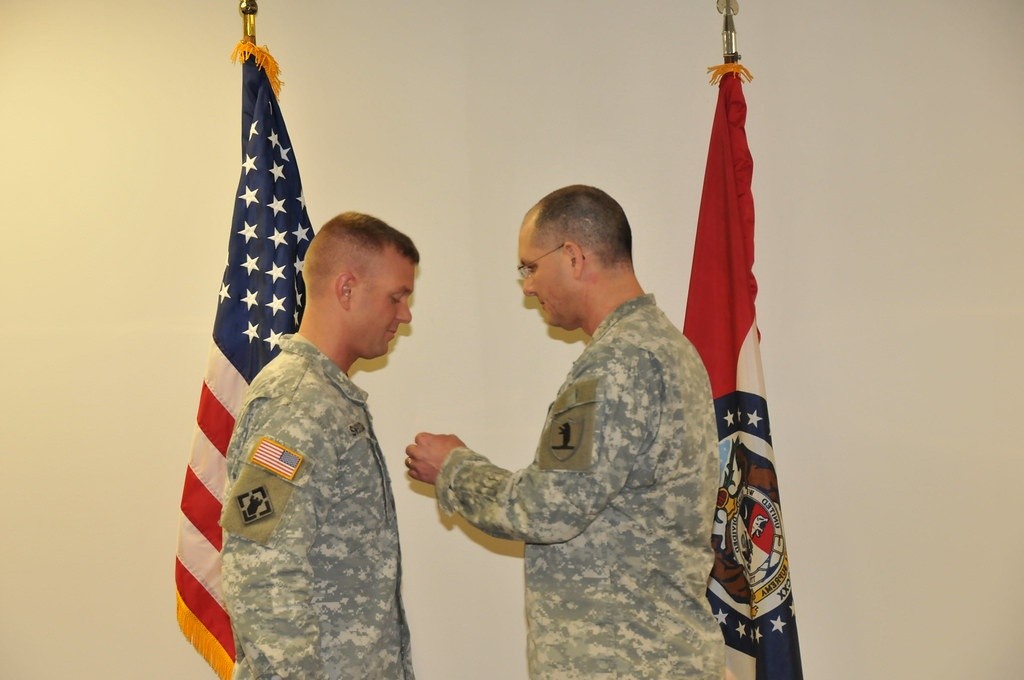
[406,457,412,468]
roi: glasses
[517,242,585,279]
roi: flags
[169,42,316,680]
[681,66,809,680]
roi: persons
[216,211,422,680]
[402,182,729,679]
